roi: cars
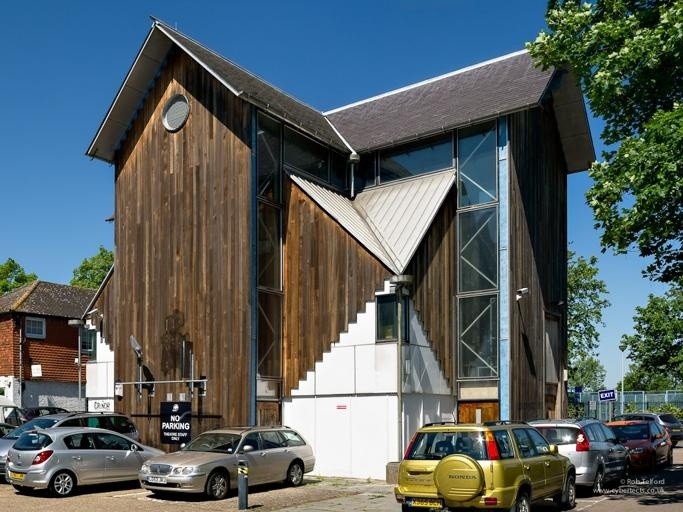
[137,424,316,499]
[0,405,167,497]
[525,411,683,497]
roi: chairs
[435,437,472,454]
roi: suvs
[394,420,576,511]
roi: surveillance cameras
[87,308,99,317]
[517,288,529,294]
[130,334,141,358]
